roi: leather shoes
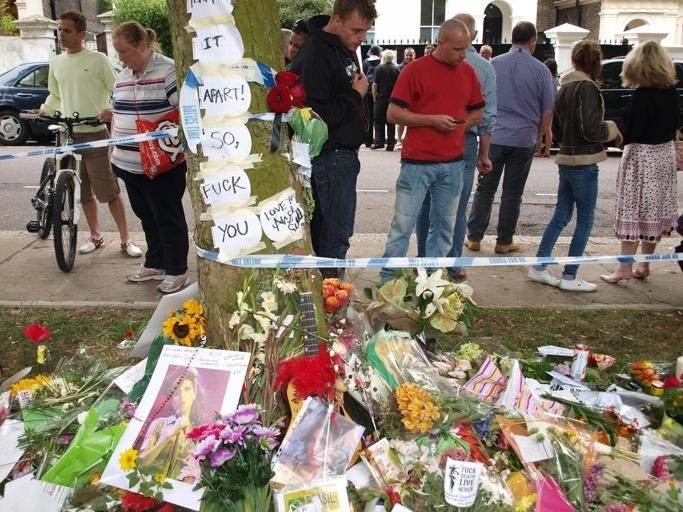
[449,269,467,284]
[386,146,393,151]
[371,144,385,150]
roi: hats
[292,18,307,36]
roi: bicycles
[25,111,101,274]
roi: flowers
[268,70,318,224]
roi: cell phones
[452,119,466,124]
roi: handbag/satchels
[135,109,187,180]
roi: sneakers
[79,234,106,254]
[527,266,560,287]
[559,276,598,292]
[127,265,166,283]
[120,240,142,257]
[463,239,481,251]
[395,143,402,150]
[156,271,191,294]
[495,240,520,254]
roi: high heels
[632,267,650,284]
[600,269,633,289]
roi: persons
[134,364,207,483]
[414,14,496,281]
[598,38,682,289]
[291,1,376,287]
[282,16,560,158]
[365,18,484,299]
[26,10,144,259]
[108,21,190,295]
[281,411,336,480]
[526,41,625,293]
[464,21,559,253]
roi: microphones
[175,411,187,436]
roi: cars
[534,58,682,159]
[0,63,56,146]
